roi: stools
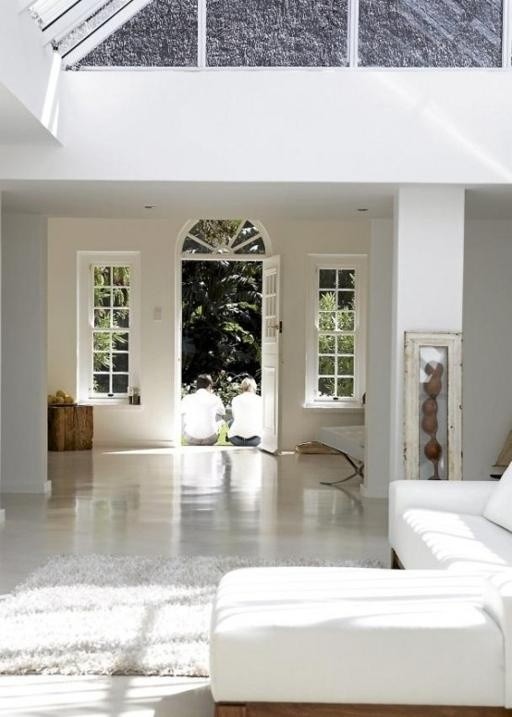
[313,424,365,487]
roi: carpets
[2,550,395,673]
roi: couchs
[211,478,511,704]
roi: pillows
[483,456,512,529]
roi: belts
[236,436,258,441]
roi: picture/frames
[406,330,464,481]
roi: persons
[226,377,262,446]
[181,375,225,446]
